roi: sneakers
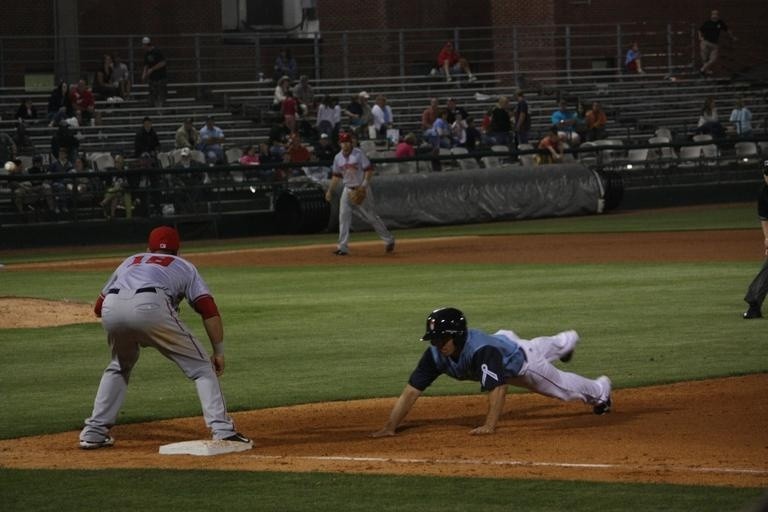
[80,436,114,447]
[594,395,610,414]
[743,309,761,318]
[218,433,254,446]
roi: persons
[625,42,644,75]
[729,100,753,141]
[76,225,255,452]
[743,161,768,319]
[366,306,613,435]
[326,134,396,255]
[697,9,739,76]
[238,140,261,182]
[697,95,718,128]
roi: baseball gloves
[349,184,367,204]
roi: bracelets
[213,342,223,356]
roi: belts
[108,287,157,293]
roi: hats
[338,133,352,142]
[149,227,179,252]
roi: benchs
[214,62,768,180]
[2,61,329,218]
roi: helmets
[420,308,467,341]
